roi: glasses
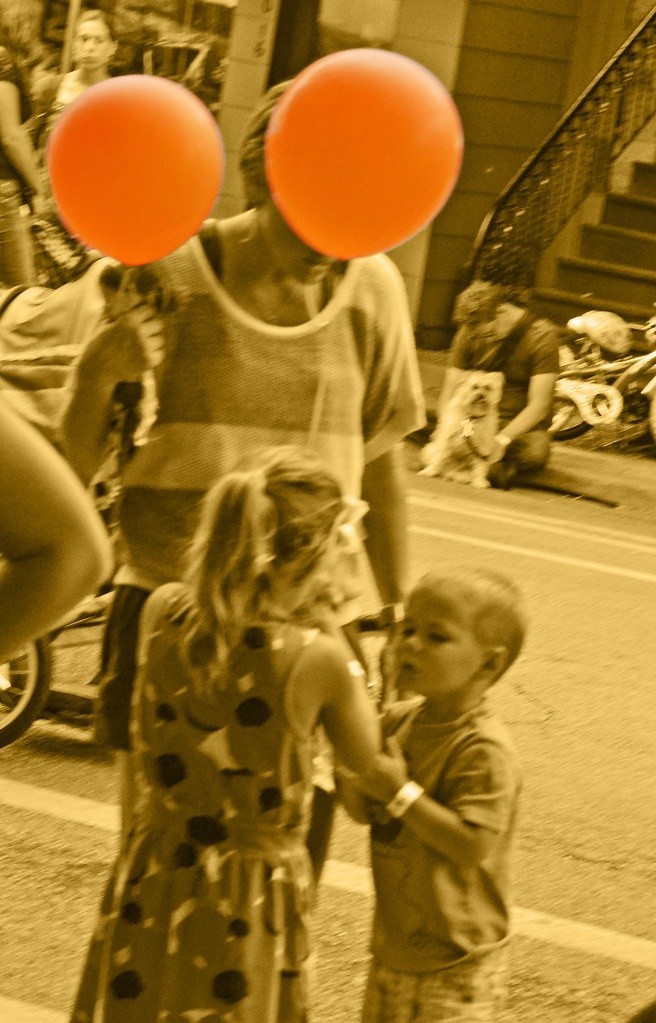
[464,318,500,340]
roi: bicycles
[545,310,655,442]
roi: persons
[0,401,115,665]
[0,0,47,287]
[55,78,433,933]
[339,563,533,1023]
[69,446,379,1020]
[37,8,119,156]
[423,279,558,492]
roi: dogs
[414,367,511,489]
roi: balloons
[266,50,467,262]
[47,72,224,269]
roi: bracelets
[386,780,424,818]
